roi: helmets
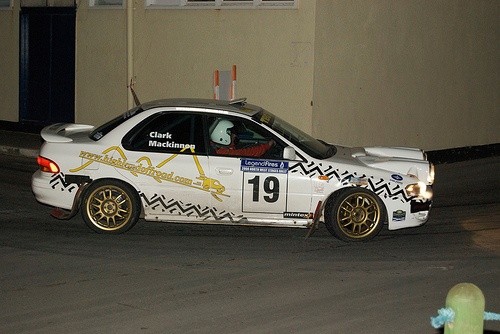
[208,117,234,145]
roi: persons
[209,118,277,157]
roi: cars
[30,98,437,244]
